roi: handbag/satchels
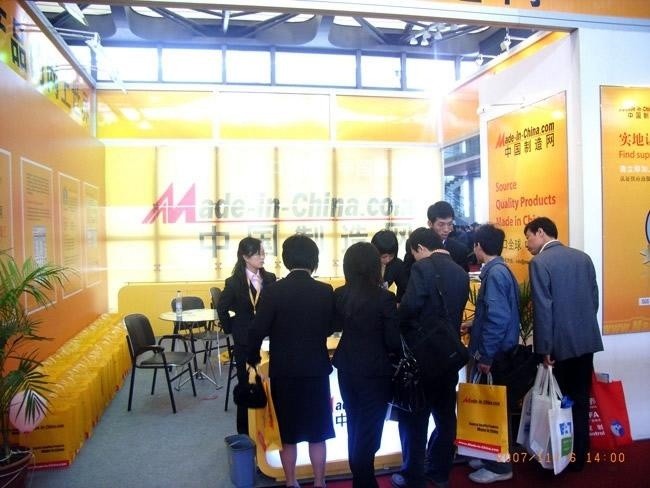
[233,365,267,407]
[416,315,469,378]
[516,362,574,474]
[456,371,511,462]
[390,333,421,388]
[589,368,633,449]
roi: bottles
[174,290,183,321]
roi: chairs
[225,333,237,411]
[169,297,226,379]
[203,287,224,364]
[124,314,196,414]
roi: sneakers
[468,459,513,482]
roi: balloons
[9,389,49,433]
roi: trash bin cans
[223,433,257,487]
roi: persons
[370,230,404,307]
[523,217,604,472]
[332,242,399,488]
[244,233,334,488]
[389,227,470,488]
[450,220,481,264]
[406,200,469,273]
[460,225,521,484]
[217,236,277,434]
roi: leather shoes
[391,470,448,487]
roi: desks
[160,309,223,390]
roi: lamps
[476,53,496,65]
[407,22,458,46]
[35,2,89,26]
[12,19,107,58]
[477,97,523,115]
[500,36,527,51]
[84,65,127,95]
